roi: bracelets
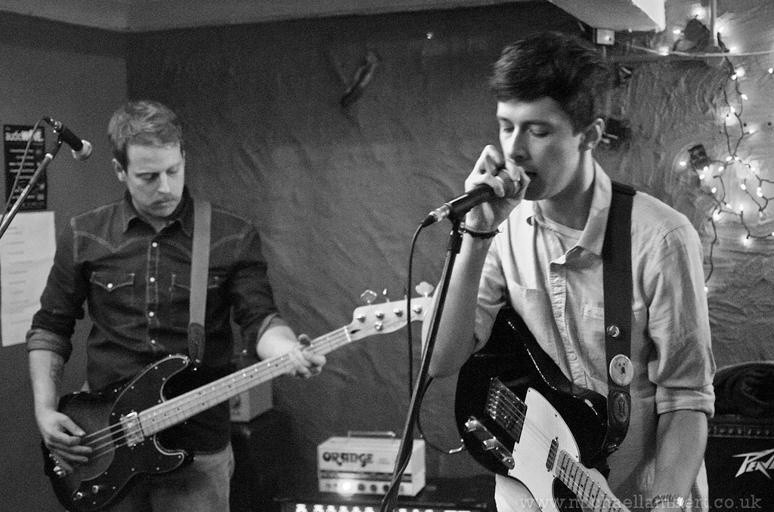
[652,494,686,512]
[462,228,499,240]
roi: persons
[26,100,328,512]
[420,30,719,512]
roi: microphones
[421,169,523,227]
[44,116,94,160]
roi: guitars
[455,306,630,510]
[43,281,434,510]
